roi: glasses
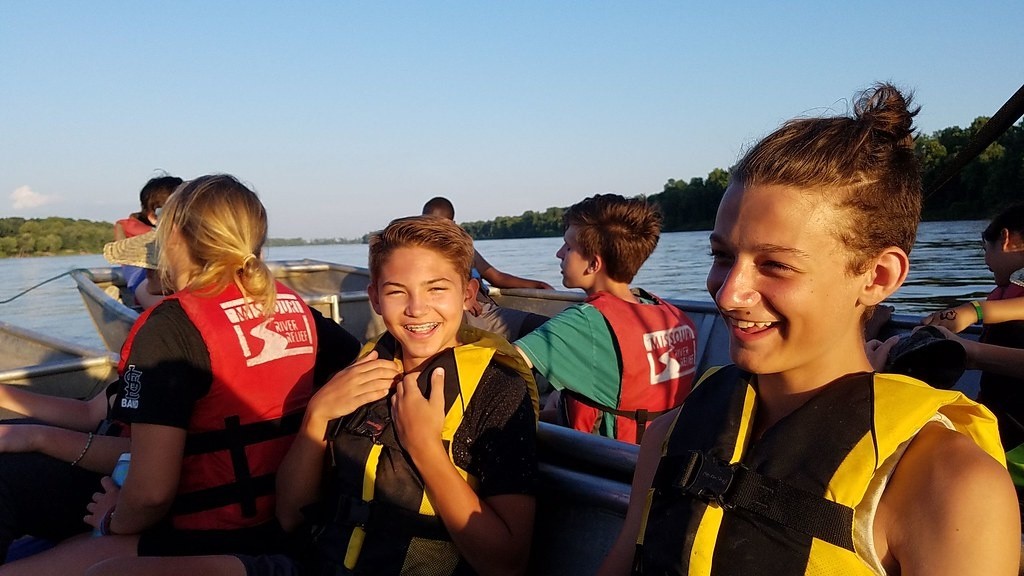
[153,207,162,216]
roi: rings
[872,338,878,349]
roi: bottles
[91,452,131,537]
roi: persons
[1,176,185,426]
[1,174,364,576]
[86,215,541,575]
[511,192,699,445]
[1,230,183,564]
[971,209,1024,452]
[423,197,556,418]
[598,79,1024,576]
[921,295,1024,334]
[864,325,1024,489]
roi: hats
[102,226,159,269]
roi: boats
[69,259,988,486]
[0,324,633,576]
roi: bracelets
[968,298,985,326]
[98,501,121,536]
[66,429,94,465]
